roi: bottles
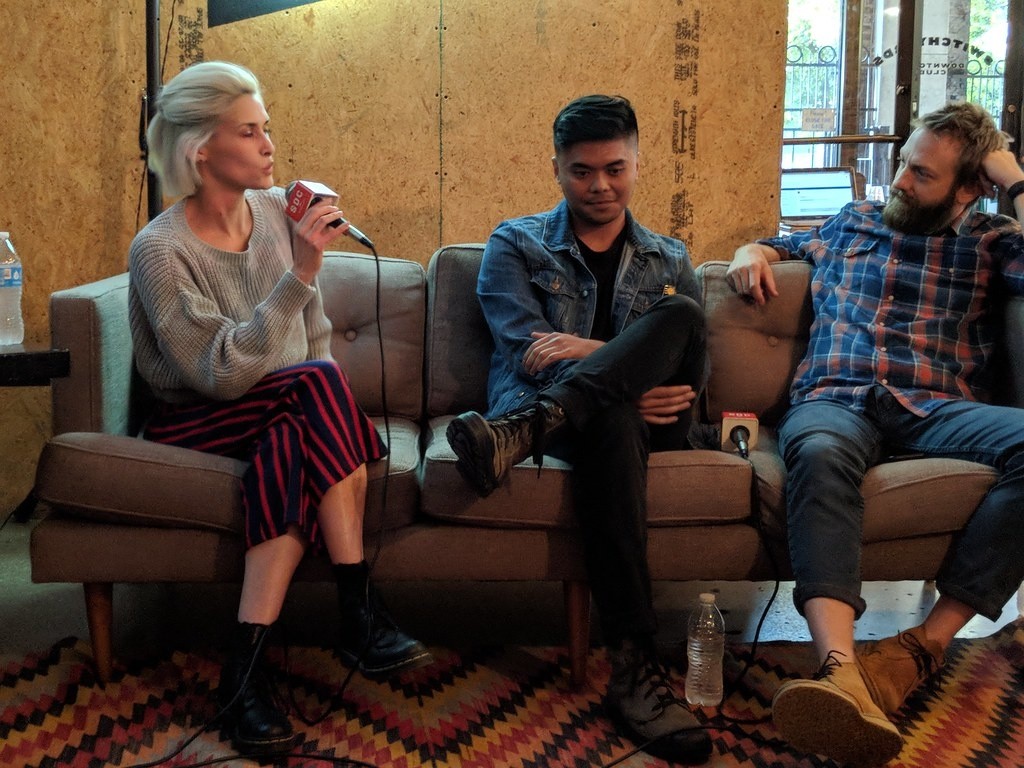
[684,592,726,707]
[0,231,25,346]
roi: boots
[216,619,296,753]
[331,559,434,680]
[602,639,713,755]
[446,394,568,497]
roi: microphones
[720,411,763,460]
[285,180,376,248]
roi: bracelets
[1006,180,1024,202]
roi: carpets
[0,613,1024,768]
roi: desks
[0,342,70,386]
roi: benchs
[28,242,1024,688]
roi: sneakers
[772,650,903,768]
[854,624,944,715]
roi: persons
[127,60,436,763]
[724,102,1024,768]
[446,94,712,766]
